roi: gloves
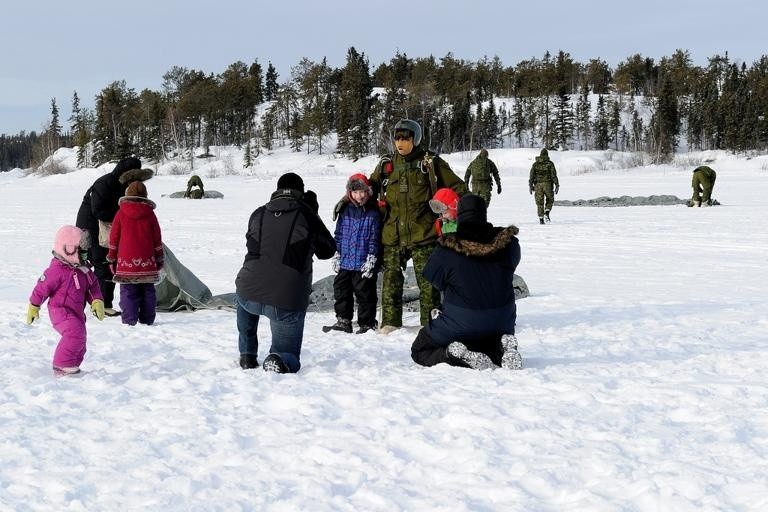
[27,303,41,325]
[109,264,116,275]
[90,299,105,322]
[359,253,379,280]
[332,199,347,221]
[331,251,342,273]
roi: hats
[346,174,373,208]
[124,181,149,197]
[455,194,488,230]
[276,173,304,193]
[429,188,459,220]
[392,119,422,147]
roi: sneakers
[443,340,496,371]
[499,333,523,370]
[139,319,153,326]
[53,363,81,374]
[239,354,259,369]
[355,320,379,334]
[104,307,122,317]
[322,317,352,334]
[262,354,288,374]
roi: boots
[544,211,551,224]
[539,218,546,224]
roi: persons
[106,181,163,325]
[529,149,559,224]
[323,173,380,334]
[411,194,522,371]
[28,226,105,374]
[235,173,336,374]
[184,175,205,198]
[429,188,461,238]
[333,119,466,335]
[692,166,716,207]
[465,149,502,208]
[76,157,153,316]
